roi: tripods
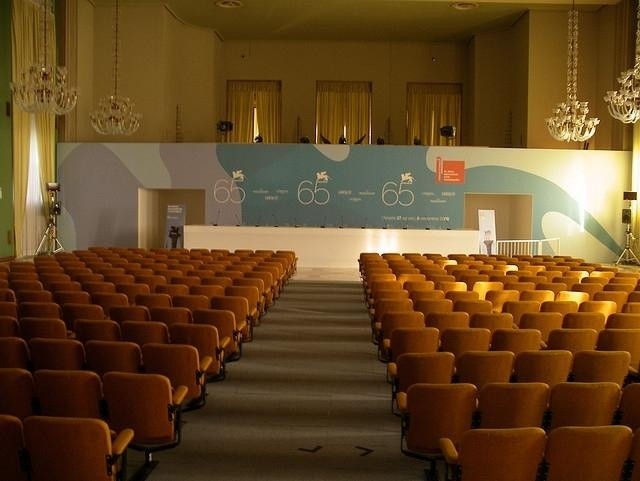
[35,215,65,255]
[615,223,640,266]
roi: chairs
[358,253,640,479]
[0,248,297,479]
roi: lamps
[88,1,143,136]
[8,0,80,116]
[545,1,601,141]
[603,0,640,124]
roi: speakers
[51,201,61,215]
[622,209,631,223]
